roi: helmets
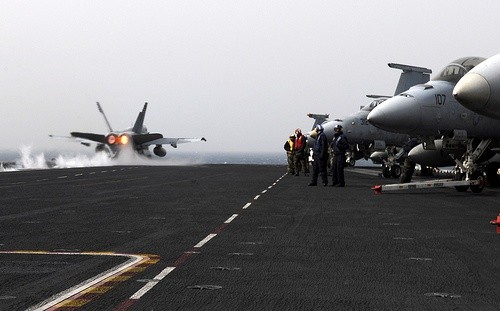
[316,125,324,131]
[289,134,296,138]
[295,128,301,134]
[334,125,342,129]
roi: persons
[284,128,309,176]
[329,125,346,187]
[308,124,328,186]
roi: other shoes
[308,182,317,186]
[330,183,336,186]
[294,172,299,176]
[336,183,345,187]
[322,181,328,186]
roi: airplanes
[304,53,500,192]
[48,102,207,161]
